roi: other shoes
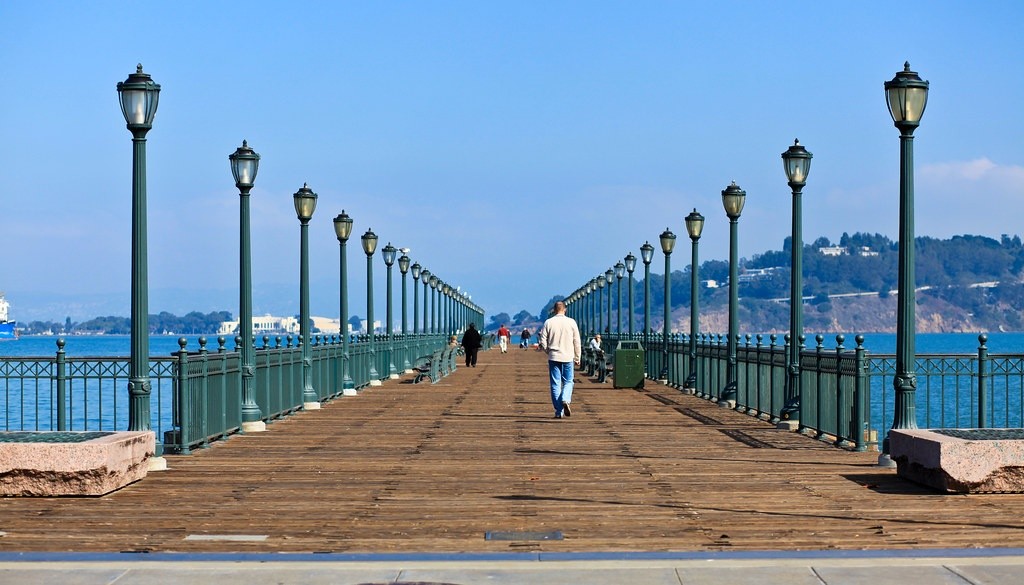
[524,348,527,351]
[466,364,470,368]
[554,414,564,419]
[473,364,476,367]
[504,351,507,353]
[562,401,571,417]
[501,352,504,354]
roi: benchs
[580,344,613,384]
[413,347,458,385]
[477,333,495,353]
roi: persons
[541,301,582,418]
[448,336,463,351]
[589,334,605,358]
[498,325,507,354]
[505,328,511,345]
[520,328,531,348]
[462,323,482,368]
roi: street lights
[640,241,657,380]
[603,268,617,337]
[360,227,383,386]
[720,180,747,408]
[547,274,607,339]
[228,139,269,434]
[291,183,326,412]
[877,58,930,466]
[381,242,400,379]
[115,64,162,451]
[781,137,815,429]
[330,208,361,399]
[411,261,485,337]
[612,260,626,336]
[685,208,706,397]
[623,252,638,347]
[398,253,414,374]
[657,227,678,384]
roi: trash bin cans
[613,340,645,389]
[585,337,595,346]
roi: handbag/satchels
[519,343,523,348]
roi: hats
[524,328,528,331]
